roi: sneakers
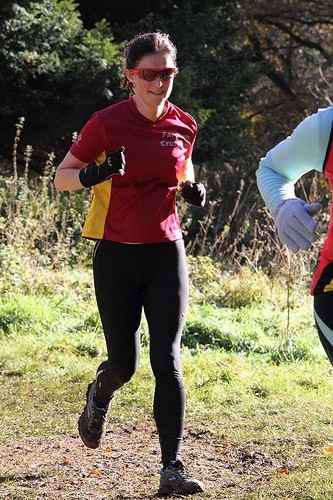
[157,458,204,496]
[78,380,115,448]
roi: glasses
[130,67,177,81]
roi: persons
[53,30,208,494]
[254,107,332,368]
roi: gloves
[182,180,206,207]
[273,198,321,254]
[78,146,126,188]
